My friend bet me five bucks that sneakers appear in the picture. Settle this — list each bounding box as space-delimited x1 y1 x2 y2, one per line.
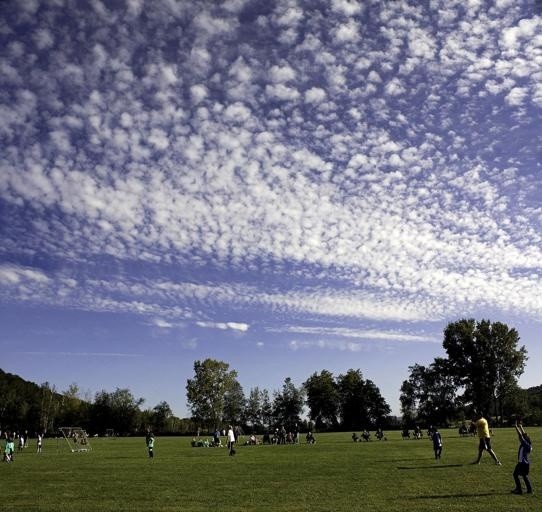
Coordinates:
511 488 523 496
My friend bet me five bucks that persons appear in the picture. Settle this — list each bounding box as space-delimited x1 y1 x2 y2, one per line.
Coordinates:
352 409 503 466
510 420 536 495
144 425 156 456
191 419 316 456
147 434 155 459
0 428 91 463
